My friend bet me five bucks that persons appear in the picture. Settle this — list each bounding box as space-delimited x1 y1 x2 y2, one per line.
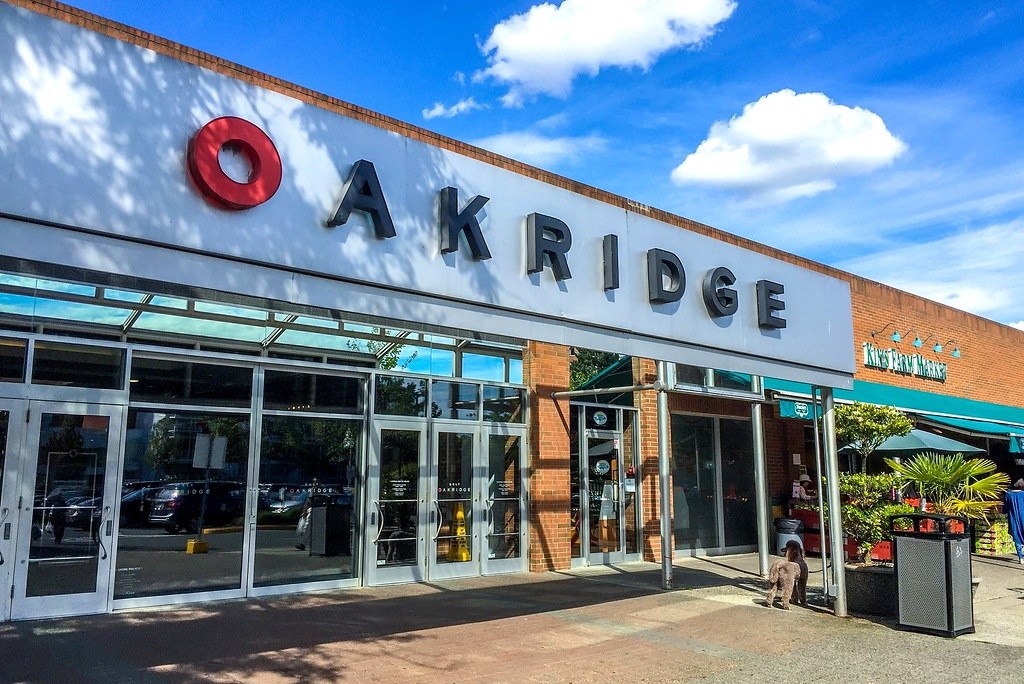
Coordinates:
798 474 818 500
1002 477 1024 564
43 488 67 545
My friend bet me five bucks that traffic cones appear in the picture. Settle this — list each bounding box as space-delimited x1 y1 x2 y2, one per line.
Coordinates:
445 501 471 562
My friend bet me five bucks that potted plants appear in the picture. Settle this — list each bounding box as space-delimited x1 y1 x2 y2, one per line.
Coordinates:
794 400 917 623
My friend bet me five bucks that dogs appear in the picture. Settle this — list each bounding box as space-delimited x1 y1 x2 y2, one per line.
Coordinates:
767 540 809 610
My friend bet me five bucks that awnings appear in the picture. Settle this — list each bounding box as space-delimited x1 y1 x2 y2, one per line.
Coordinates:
712 370 1024 454
573 355 638 448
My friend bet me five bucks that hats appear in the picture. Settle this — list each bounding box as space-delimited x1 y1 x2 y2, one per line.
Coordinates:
799 475 813 483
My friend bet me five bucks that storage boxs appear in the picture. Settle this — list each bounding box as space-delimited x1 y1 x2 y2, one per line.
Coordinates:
974 513 1024 557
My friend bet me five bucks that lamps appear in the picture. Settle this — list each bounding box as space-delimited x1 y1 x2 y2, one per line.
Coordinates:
941 340 961 358
872 323 901 343
922 334 942 353
894 329 923 349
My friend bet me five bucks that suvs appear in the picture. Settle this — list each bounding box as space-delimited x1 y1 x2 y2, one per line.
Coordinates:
147 479 270 534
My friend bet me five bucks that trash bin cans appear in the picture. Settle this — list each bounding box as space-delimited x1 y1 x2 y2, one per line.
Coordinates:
889 513 976 640
774 517 805 557
311 492 352 557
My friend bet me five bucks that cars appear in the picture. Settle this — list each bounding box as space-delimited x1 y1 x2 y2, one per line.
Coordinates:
260 482 355 527
31 479 172 529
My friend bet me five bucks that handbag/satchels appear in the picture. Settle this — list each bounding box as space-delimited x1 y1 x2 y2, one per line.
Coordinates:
297 516 306 536
45 521 53 533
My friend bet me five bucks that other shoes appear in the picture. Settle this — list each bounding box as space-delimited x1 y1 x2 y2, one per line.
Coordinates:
296 543 305 551
55 538 61 544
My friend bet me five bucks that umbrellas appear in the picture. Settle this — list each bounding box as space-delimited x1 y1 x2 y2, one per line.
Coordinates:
837 428 988 477
570 440 617 464
